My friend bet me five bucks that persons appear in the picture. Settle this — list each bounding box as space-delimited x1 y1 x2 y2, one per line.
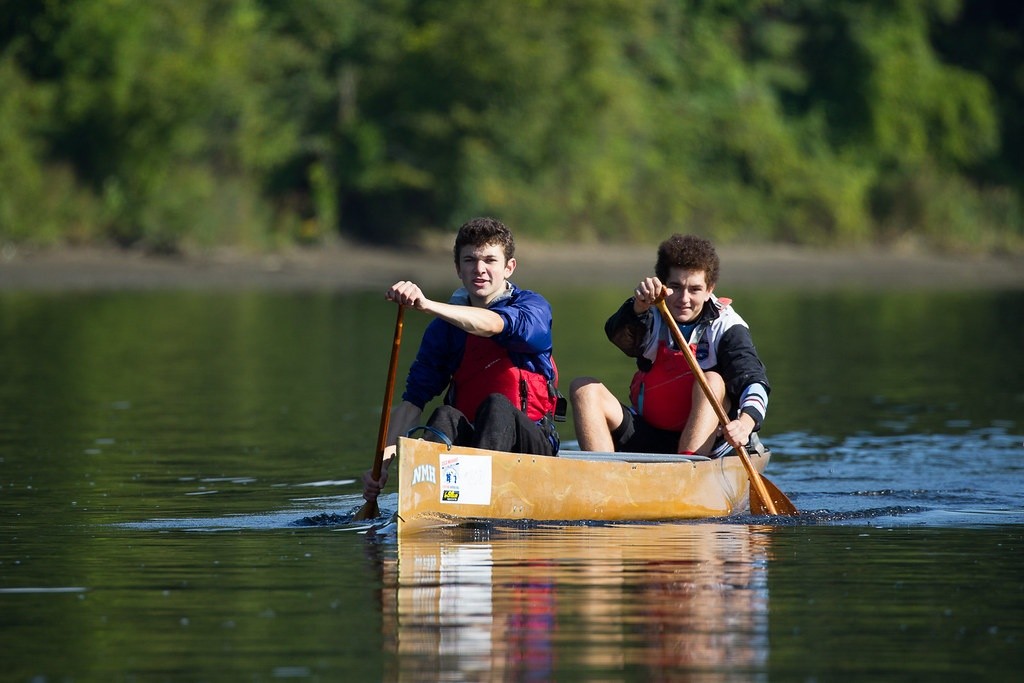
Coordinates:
361 218 561 503
568 233 771 462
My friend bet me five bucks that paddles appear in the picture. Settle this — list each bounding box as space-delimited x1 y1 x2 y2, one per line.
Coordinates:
647 285 798 514
354 298 404 518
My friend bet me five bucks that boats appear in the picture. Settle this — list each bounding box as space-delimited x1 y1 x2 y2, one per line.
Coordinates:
394 423 773 535
